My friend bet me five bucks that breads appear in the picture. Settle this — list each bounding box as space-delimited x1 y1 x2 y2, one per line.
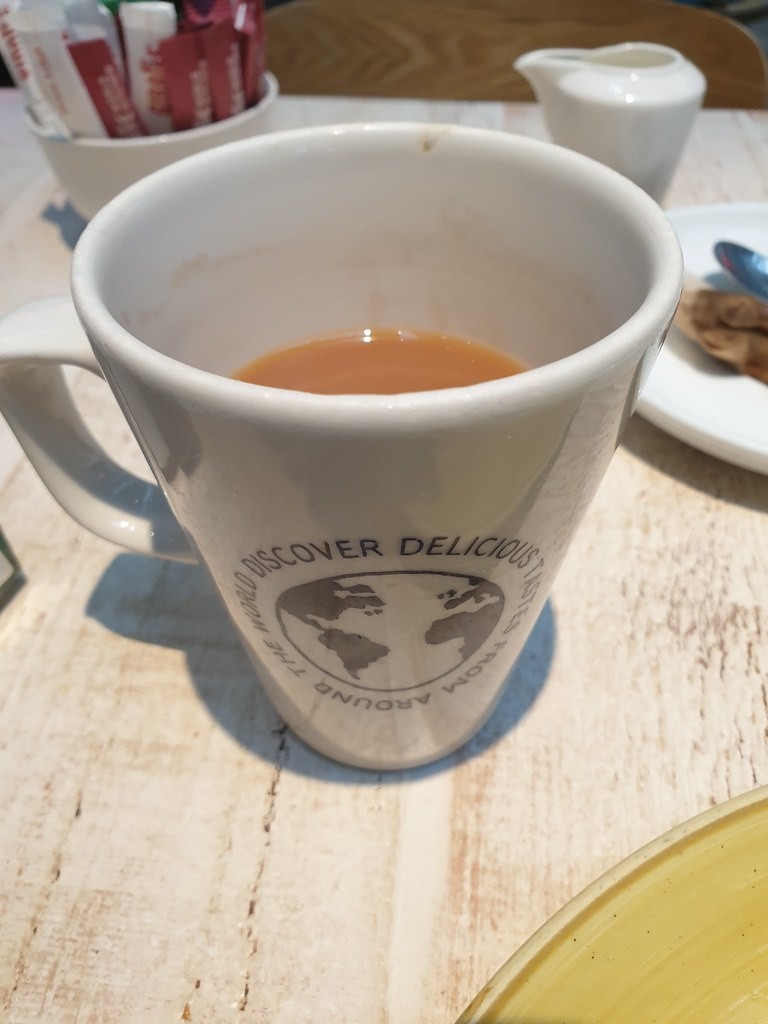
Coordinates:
672 276 768 382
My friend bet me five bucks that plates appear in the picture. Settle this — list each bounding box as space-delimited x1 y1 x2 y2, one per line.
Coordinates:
453 785 768 1024
635 200 768 476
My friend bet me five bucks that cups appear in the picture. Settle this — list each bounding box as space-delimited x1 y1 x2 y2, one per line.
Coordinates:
0 122 685 771
514 43 707 201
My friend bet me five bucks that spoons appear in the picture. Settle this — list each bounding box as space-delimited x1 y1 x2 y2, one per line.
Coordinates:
714 242 768 304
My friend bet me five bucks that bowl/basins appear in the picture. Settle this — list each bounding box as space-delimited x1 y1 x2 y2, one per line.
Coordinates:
22 71 279 221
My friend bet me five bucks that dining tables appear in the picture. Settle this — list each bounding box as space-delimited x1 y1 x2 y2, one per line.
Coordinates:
0 88 768 1024
